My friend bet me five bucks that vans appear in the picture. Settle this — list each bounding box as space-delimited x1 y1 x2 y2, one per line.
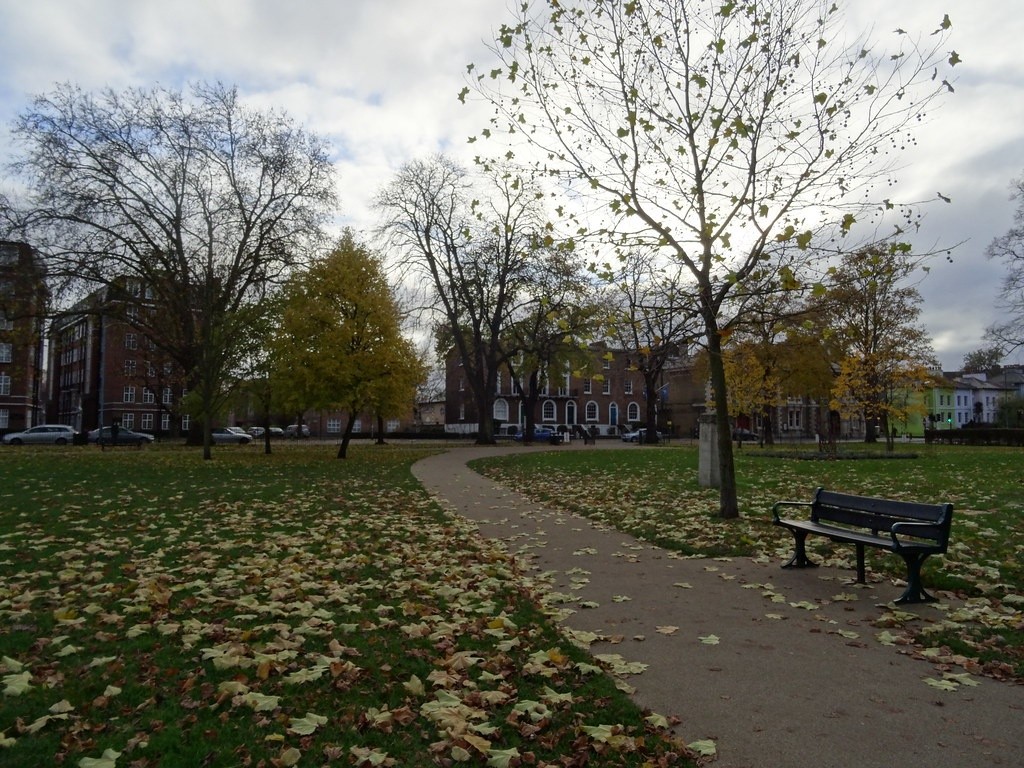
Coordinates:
284 424 311 438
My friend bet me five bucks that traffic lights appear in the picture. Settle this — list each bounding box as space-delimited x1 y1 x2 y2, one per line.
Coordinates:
947 412 952 423
929 412 933 421
937 413 941 421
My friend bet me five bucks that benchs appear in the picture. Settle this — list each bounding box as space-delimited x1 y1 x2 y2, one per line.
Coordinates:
775 487 956 605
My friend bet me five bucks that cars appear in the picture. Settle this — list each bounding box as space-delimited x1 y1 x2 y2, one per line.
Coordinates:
270 425 284 436
732 427 760 440
225 427 245 434
248 426 265 438
621 428 662 443
3 423 80 445
88 425 156 446
514 425 552 441
210 427 253 445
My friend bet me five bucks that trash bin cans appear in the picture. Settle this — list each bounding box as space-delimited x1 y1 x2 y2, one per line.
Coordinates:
639 429 646 444
